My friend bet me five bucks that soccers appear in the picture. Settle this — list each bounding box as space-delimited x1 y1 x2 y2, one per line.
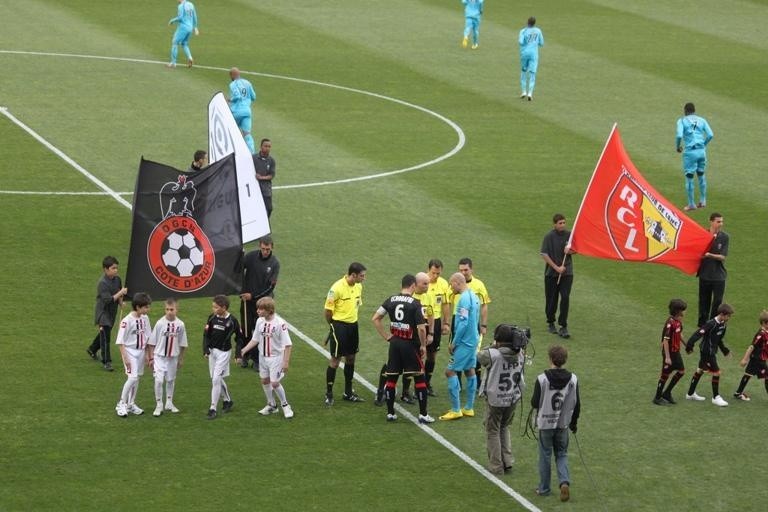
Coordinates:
161 229 205 278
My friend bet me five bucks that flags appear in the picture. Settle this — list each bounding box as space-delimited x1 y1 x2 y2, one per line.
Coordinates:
568 127 713 275
123 157 246 302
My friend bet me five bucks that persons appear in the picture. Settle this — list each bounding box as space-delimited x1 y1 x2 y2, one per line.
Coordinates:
477 323 526 473
539 214 577 338
696 213 730 328
461 0 484 50
164 0 200 68
324 262 368 406
88 235 294 419
518 17 544 100
652 300 768 407
531 345 581 502
227 68 256 154
252 139 275 218
185 150 208 228
674 103 712 211
371 258 492 424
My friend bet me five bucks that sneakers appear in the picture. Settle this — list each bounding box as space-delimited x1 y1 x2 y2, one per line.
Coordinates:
684 202 705 211
560 482 569 501
165 60 194 69
462 36 478 49
519 92 532 100
342 389 362 401
206 408 216 419
547 322 570 338
103 361 113 370
375 383 484 424
652 392 750 406
281 402 295 417
85 347 97 359
222 399 234 411
324 393 334 404
259 404 279 416
114 400 180 418
